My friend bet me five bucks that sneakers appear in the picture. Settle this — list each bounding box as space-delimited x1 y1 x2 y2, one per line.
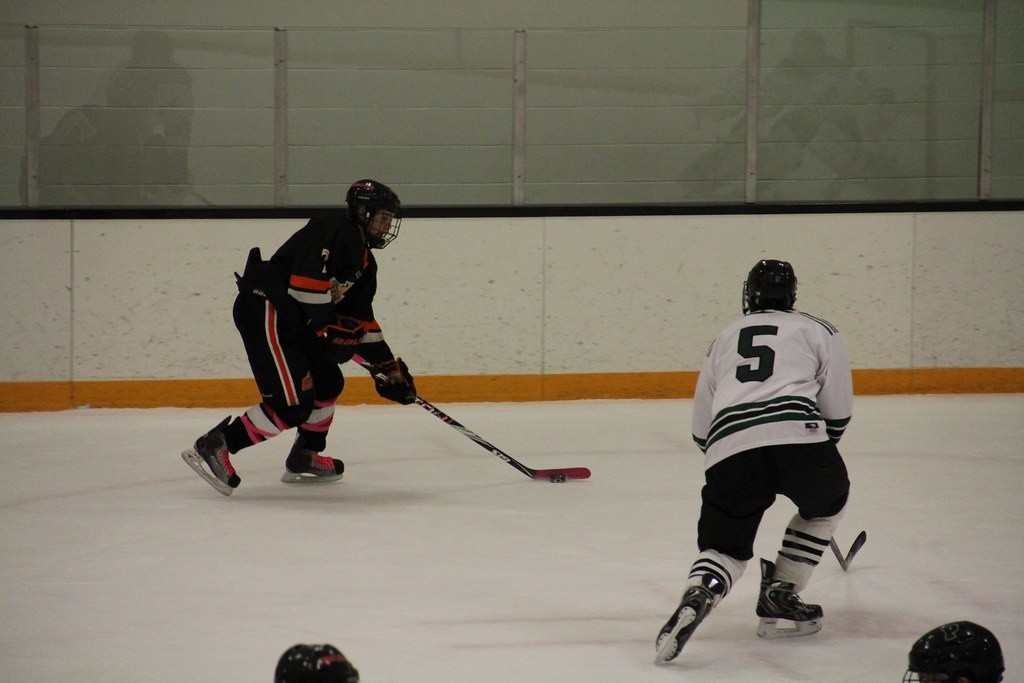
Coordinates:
756 557 824 640
653 572 728 665
281 448 345 483
181 415 241 496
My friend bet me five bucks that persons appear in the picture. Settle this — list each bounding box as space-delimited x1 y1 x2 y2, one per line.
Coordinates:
902 621 1006 683
652 259 853 658
180 178 417 493
272 643 360 683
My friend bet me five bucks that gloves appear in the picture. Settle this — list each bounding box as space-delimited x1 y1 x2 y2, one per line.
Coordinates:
316 318 367 363
375 357 416 405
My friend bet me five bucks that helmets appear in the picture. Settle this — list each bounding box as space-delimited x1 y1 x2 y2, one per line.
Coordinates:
345 179 403 249
742 259 798 315
902 621 1006 683
274 643 359 683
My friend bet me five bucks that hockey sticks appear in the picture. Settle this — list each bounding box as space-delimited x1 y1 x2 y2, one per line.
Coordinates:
829 530 867 572
351 353 592 480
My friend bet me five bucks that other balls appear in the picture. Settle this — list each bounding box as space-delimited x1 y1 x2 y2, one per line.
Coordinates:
551 473 565 484
